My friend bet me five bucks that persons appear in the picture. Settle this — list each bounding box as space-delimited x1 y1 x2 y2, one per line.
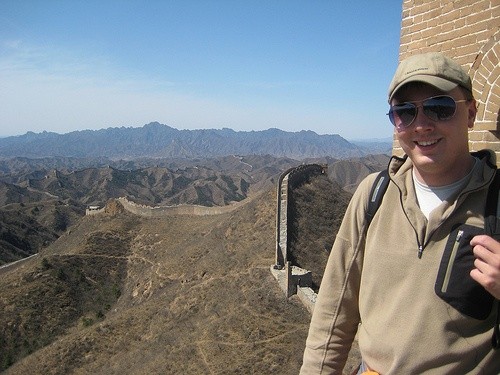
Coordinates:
298 52 500 375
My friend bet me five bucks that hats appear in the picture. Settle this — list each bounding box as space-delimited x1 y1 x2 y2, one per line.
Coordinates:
387 52 473 103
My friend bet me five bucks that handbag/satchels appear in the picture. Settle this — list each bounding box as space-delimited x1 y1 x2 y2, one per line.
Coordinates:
433 224 493 319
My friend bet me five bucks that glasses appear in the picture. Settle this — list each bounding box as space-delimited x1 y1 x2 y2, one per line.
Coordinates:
388 95 456 128
